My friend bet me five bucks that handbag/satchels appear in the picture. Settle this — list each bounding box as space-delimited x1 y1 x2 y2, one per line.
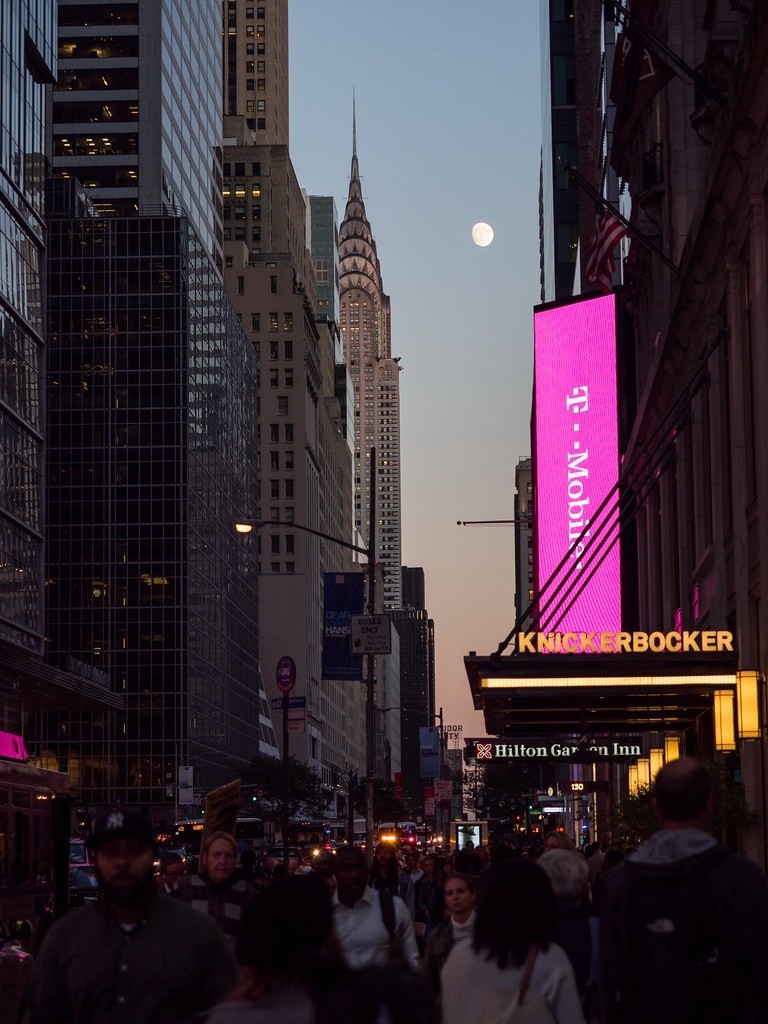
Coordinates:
483 943 555 1024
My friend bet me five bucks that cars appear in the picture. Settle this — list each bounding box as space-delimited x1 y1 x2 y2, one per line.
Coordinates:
0 798 453 1024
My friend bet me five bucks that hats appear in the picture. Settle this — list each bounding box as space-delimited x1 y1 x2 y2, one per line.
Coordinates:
86 808 155 848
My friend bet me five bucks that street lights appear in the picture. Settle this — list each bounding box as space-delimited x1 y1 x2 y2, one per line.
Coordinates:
229 446 379 868
376 707 444 832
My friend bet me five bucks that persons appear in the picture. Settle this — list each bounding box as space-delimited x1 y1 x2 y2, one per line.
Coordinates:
598 757 768 1024
27 808 237 1023
157 828 631 1024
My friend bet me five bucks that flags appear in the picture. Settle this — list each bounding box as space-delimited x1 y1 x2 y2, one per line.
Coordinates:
583 200 631 295
609 27 679 208
